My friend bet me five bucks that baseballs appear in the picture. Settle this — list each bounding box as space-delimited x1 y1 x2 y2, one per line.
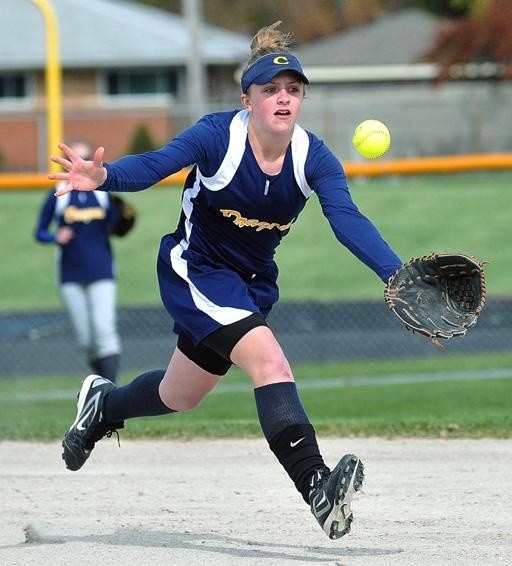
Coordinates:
353 119 391 158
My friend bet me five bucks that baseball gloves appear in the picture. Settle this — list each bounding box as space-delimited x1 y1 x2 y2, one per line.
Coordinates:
386 253 486 339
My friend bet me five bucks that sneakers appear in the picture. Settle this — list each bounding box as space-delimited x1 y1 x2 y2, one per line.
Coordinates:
61 373 125 472
307 454 366 540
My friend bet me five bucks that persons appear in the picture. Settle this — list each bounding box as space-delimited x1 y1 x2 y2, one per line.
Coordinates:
47 20 486 541
34 139 137 386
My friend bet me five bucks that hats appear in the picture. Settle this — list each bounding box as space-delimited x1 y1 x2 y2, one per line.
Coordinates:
239 51 309 95
